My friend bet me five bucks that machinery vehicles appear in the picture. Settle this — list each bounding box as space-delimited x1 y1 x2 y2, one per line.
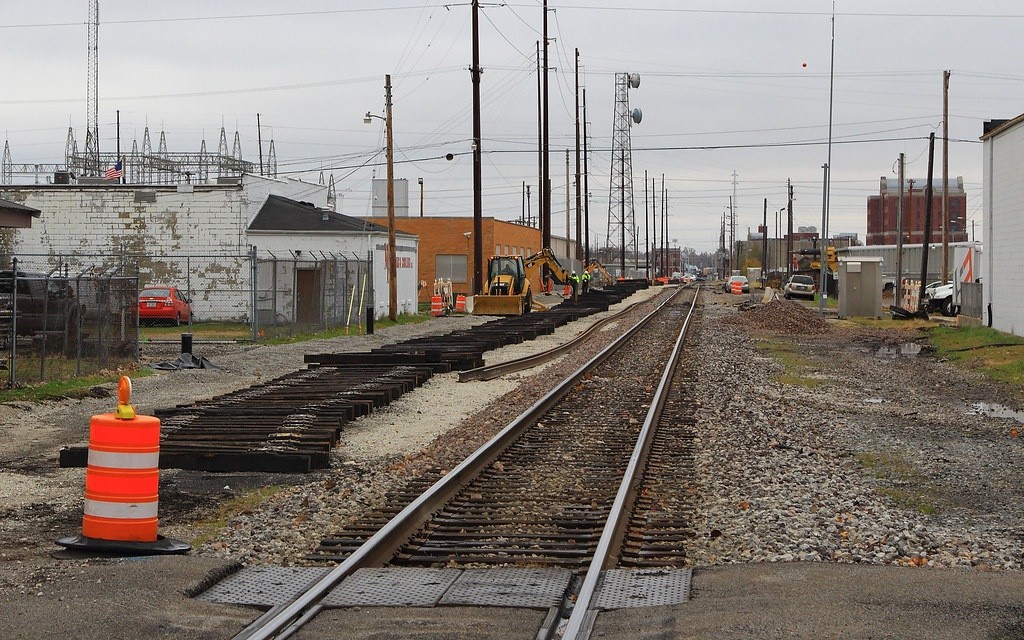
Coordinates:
470 247 570 316
584 258 614 284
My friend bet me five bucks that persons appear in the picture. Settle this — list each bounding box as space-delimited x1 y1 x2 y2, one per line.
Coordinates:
582 271 591 292
793 258 798 271
570 271 580 283
503 264 514 274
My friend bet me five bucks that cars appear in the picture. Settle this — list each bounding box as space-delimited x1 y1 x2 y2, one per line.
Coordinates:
725 276 750 293
924 280 961 317
783 275 816 301
130 284 194 325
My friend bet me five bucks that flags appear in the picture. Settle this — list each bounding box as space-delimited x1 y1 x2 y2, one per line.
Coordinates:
104 160 122 180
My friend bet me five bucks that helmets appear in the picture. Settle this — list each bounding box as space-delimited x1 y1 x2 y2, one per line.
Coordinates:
584 271 588 274
572 271 575 274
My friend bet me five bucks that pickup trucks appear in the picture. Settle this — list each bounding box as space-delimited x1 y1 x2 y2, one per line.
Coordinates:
0 270 86 350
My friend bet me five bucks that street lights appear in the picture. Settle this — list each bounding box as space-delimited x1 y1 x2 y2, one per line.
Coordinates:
566 191 593 281
774 208 786 278
363 74 398 323
